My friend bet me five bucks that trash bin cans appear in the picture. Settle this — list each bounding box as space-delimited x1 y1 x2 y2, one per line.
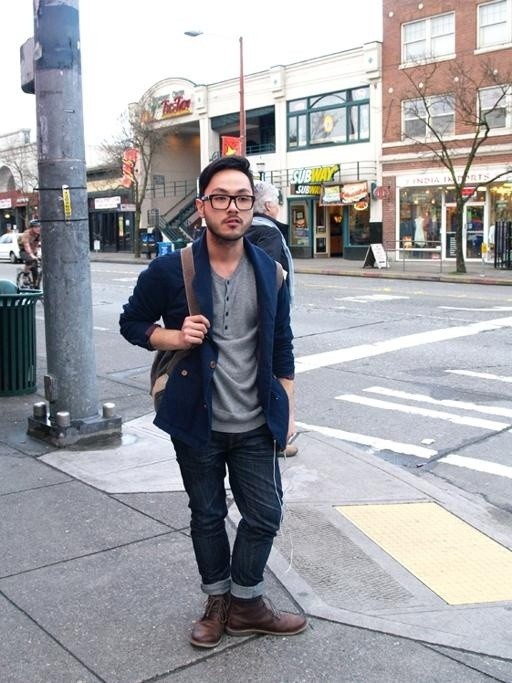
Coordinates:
0 279 45 397
157 241 187 257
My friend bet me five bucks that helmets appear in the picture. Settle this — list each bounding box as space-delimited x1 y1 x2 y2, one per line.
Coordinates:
29 218 40 227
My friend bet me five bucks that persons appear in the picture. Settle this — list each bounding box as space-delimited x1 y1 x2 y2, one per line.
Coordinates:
16 217 41 287
112 154 311 648
243 178 294 300
411 215 427 247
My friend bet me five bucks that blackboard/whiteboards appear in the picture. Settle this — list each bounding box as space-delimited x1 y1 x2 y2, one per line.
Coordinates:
371 244 389 267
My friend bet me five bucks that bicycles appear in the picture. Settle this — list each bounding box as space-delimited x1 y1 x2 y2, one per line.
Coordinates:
16 257 44 304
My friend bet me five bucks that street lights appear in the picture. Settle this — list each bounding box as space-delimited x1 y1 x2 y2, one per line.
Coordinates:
183 29 246 159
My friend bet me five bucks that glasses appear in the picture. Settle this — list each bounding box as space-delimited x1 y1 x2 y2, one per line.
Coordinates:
201 193 255 211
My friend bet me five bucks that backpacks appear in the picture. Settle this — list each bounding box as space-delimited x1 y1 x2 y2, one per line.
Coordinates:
150 245 284 411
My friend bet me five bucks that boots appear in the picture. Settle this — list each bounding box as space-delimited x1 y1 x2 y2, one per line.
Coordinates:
225 594 309 636
190 590 230 648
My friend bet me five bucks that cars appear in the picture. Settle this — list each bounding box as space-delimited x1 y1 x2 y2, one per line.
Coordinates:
0 232 42 264
